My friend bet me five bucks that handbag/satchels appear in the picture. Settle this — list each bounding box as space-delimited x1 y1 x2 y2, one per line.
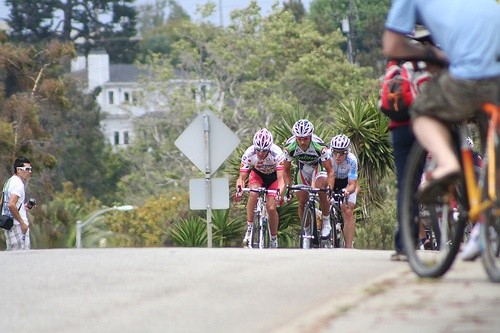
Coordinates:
0 215 13 230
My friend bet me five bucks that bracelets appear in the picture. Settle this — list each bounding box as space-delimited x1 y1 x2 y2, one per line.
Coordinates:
424 41 436 62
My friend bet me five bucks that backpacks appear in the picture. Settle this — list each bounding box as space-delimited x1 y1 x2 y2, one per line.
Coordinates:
378 59 433 121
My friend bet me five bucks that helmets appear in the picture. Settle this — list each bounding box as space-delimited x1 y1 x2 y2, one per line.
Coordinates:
291 119 314 137
252 128 273 150
331 133 350 151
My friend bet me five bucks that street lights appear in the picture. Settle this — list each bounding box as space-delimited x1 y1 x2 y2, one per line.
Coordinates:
75 205 133 249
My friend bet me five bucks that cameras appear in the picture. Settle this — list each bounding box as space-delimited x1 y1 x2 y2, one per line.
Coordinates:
27 199 36 209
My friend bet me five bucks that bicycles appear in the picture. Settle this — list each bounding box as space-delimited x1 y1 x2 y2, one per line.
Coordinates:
236 186 278 249
397 53 500 282
286 184 349 250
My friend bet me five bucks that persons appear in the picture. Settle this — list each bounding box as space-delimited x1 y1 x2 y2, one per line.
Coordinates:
282 119 334 249
377 0 500 261
327 133 360 249
0 156 37 251
235 127 286 249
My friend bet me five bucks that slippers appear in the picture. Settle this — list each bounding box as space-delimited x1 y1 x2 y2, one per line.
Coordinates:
415 169 461 203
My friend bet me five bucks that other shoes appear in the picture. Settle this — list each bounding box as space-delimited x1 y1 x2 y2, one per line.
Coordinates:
389 251 407 261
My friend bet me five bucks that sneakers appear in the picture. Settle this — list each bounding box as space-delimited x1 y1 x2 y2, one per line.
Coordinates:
271 235 279 248
459 227 497 262
243 227 252 244
321 224 331 237
302 237 310 248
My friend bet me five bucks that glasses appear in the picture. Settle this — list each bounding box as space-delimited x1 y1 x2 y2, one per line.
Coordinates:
332 150 348 155
256 149 268 153
296 138 310 141
17 167 32 171
